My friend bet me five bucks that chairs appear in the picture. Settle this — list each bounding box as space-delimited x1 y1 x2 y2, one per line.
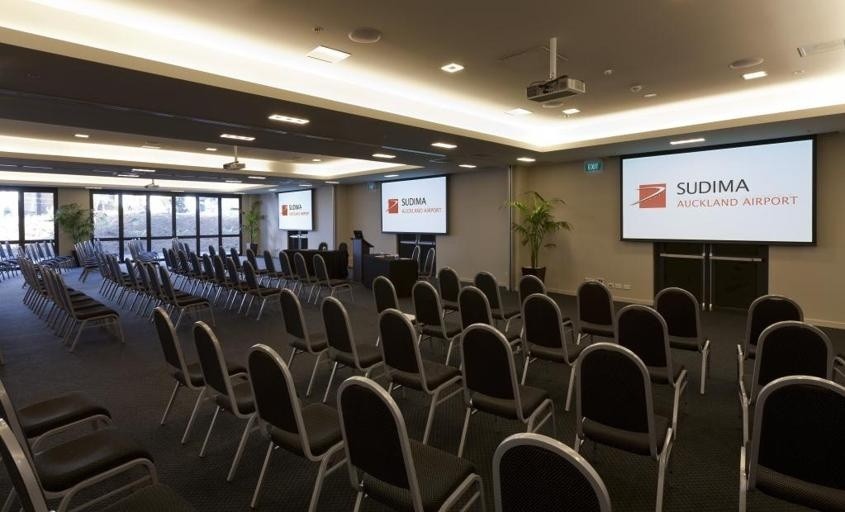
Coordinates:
189 317 279 483
333 371 489 509
566 341 679 509
278 263 844 469
2 233 358 353
736 373 843 512
490 429 614 507
0 370 170 512
150 303 249 446
242 341 347 509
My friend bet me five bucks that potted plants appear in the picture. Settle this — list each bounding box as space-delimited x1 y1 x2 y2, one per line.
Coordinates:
231 200 269 257
49 201 99 267
501 190 574 284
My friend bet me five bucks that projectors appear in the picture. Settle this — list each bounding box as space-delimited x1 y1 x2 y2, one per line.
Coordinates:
526 78 585 103
145 185 159 190
223 162 246 171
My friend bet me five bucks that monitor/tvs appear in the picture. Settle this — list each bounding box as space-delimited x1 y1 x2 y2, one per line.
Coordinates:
354 230 363 239
